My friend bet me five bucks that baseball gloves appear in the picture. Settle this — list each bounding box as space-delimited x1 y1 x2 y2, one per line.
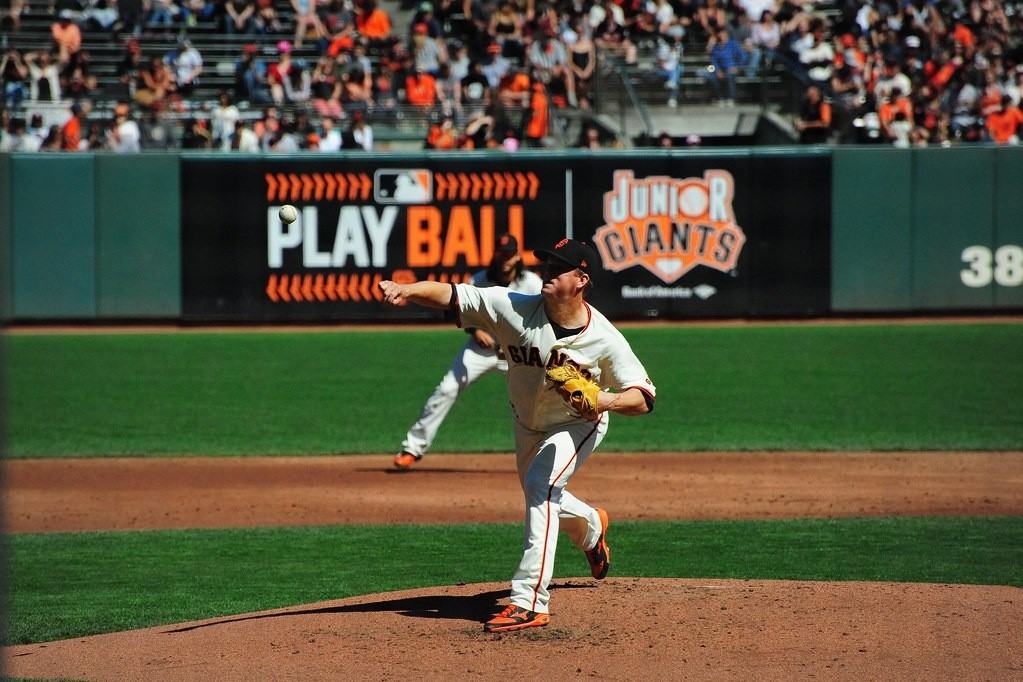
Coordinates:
546 363 602 423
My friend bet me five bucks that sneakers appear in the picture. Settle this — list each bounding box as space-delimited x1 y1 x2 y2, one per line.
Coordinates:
484 604 550 633
394 450 415 469
584 508 610 580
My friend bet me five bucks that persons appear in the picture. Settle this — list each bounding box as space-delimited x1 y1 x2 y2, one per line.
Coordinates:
378 239 658 632
0 0 1023 153
393 234 545 468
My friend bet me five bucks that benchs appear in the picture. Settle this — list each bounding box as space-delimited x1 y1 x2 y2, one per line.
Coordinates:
0 0 801 140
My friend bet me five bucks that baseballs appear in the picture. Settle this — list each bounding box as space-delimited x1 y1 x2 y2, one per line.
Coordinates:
279 205 298 224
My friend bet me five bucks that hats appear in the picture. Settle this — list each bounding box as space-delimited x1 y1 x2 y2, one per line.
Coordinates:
534 239 597 282
495 234 518 252
